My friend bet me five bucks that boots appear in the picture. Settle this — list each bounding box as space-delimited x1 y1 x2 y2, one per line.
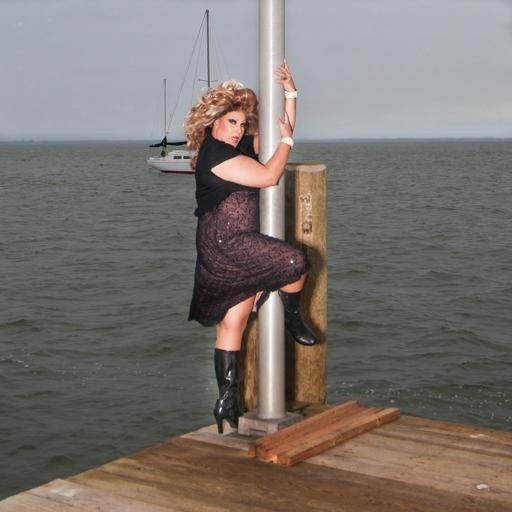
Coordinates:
280 288 318 346
212 347 246 435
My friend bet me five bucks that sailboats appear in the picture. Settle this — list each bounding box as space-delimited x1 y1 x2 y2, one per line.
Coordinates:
147 9 218 176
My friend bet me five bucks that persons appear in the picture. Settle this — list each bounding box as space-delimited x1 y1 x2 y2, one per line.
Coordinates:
183 60 318 435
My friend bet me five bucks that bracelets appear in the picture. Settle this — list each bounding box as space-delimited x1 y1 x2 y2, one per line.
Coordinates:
284 90 298 100
281 137 295 146
